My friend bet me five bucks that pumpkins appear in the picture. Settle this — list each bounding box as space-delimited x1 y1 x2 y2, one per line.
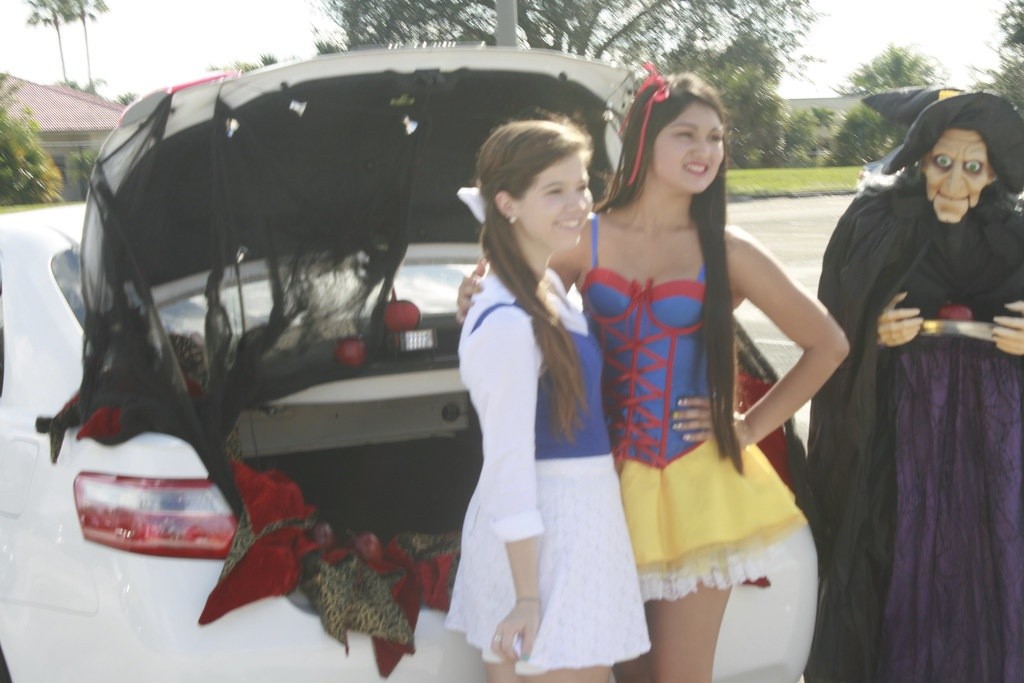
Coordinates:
385 289 420 332
336 335 369 368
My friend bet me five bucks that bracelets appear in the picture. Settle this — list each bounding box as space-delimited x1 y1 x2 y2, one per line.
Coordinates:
516 597 541 604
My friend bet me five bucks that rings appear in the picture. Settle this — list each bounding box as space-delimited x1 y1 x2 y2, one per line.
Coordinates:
493 635 502 642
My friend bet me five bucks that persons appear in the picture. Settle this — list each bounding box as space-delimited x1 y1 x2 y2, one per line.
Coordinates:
455 71 849 683
440 120 652 683
801 85 1024 683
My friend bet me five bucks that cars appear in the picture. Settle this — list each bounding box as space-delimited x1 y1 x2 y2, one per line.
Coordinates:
858 141 907 194
0 47 812 682
